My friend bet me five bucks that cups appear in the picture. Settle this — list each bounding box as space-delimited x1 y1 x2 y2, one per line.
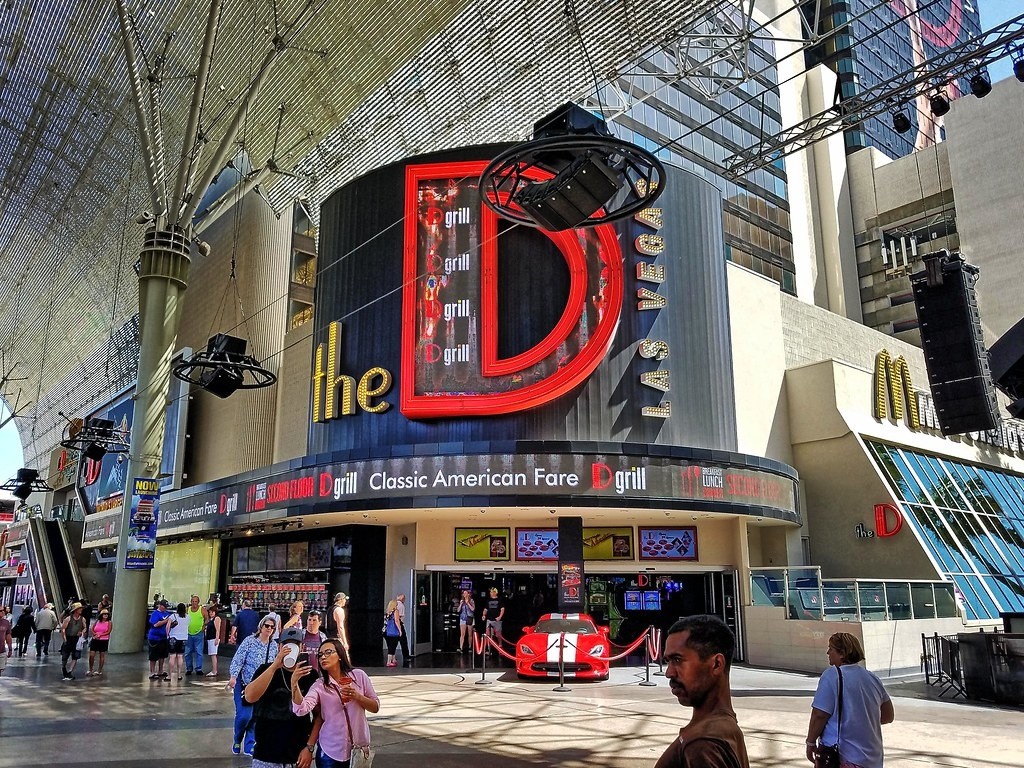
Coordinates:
282 643 299 668
339 677 352 703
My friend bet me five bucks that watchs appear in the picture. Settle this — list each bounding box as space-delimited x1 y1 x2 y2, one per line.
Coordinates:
306 743 314 753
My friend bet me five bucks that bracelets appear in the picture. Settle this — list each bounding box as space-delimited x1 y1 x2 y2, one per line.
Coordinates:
9 649 12 651
806 739 816 747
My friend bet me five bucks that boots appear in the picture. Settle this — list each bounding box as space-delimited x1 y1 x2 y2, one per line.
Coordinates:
386 654 396 666
391 655 398 664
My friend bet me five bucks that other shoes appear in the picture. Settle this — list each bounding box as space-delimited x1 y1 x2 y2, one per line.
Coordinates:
62 674 76 681
36 651 48 657
85 670 103 675
485 649 492 655
499 647 505 655
156 672 168 677
206 671 217 677
185 671 204 676
149 673 159 679
457 648 463 653
402 655 415 660
469 648 472 652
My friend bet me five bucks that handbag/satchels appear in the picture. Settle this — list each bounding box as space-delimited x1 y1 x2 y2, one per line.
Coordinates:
814 744 841 768
170 613 178 628
76 632 85 650
466 616 474 626
387 620 400 637
349 744 375 768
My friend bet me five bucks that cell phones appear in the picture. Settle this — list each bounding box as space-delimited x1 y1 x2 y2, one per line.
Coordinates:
299 652 310 667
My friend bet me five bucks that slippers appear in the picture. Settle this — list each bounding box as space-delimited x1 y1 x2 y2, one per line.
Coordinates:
178 676 182 680
163 676 171 681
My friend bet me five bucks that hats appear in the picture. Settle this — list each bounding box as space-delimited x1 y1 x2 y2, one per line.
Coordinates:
100 609 110 615
160 599 171 607
279 628 302 641
102 594 109 598
334 592 350 602
70 602 84 613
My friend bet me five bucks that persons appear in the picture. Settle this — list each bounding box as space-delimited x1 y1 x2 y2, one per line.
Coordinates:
257 602 281 653
14 605 39 658
282 601 304 630
506 586 518 610
532 588 544 608
147 593 222 680
33 603 62 656
654 615 750 768
448 586 475 652
0 604 14 677
482 586 505 655
245 628 325 768
290 638 381 768
384 593 414 667
302 610 329 676
60 594 113 678
325 592 351 666
807 632 894 768
227 616 279 756
230 600 259 650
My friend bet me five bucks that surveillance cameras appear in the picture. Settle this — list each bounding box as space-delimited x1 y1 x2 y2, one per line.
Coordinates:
118 456 124 464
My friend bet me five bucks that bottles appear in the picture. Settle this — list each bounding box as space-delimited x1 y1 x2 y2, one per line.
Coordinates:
422 595 425 604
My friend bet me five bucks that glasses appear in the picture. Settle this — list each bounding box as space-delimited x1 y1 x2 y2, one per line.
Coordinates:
0 609 5 612
264 623 275 629
318 649 338 658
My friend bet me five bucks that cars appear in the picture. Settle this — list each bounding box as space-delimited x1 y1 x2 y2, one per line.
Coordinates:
515 613 610 680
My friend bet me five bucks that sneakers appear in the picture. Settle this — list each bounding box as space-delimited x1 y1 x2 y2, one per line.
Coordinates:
231 741 254 757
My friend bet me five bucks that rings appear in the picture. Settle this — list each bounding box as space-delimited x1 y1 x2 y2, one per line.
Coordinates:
349 692 352 696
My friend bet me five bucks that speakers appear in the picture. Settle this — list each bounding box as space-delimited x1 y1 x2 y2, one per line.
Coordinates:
13 469 38 501
200 334 246 400
512 102 625 232
82 419 116 462
910 263 1003 437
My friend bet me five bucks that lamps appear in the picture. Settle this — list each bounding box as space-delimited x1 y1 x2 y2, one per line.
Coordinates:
890 240 898 271
103 548 107 555
260 523 266 533
477 0 668 235
177 538 183 543
170 251 276 400
900 236 909 267
200 532 206 541
156 540 163 547
882 247 889 265
929 86 951 118
297 518 304 529
970 66 993 99
911 236 918 257
0 427 55 500
90 548 95 555
892 105 912 134
228 528 234 537
168 539 171 544
190 534 194 542
113 545 117 552
57 360 131 462
1013 48 1024 83
281 520 288 530
985 316 1024 421
215 530 221 539
246 525 253 535
